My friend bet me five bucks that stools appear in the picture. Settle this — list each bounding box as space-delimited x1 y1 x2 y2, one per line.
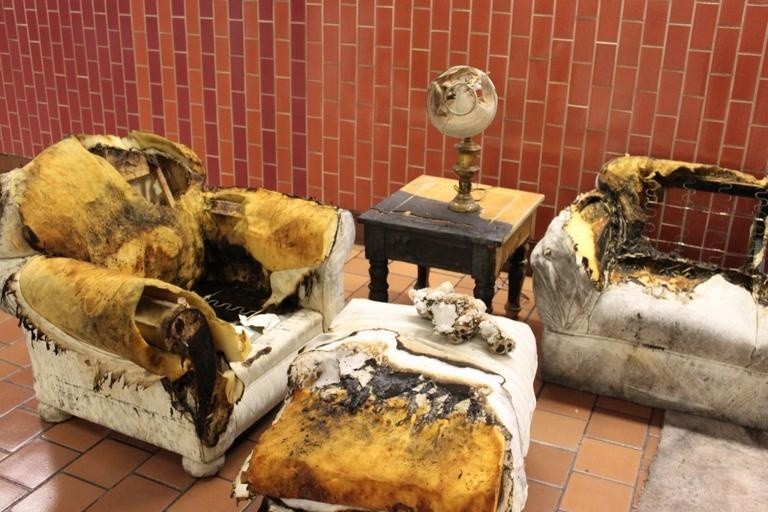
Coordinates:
234 298 540 510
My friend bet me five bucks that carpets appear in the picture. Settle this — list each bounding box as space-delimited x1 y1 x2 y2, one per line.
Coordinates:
629 410 767 510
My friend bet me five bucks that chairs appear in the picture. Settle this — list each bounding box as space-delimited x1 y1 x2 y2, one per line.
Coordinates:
0 131 357 477
529 154 767 437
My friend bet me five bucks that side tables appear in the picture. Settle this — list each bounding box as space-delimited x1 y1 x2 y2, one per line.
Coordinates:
357 171 546 316
